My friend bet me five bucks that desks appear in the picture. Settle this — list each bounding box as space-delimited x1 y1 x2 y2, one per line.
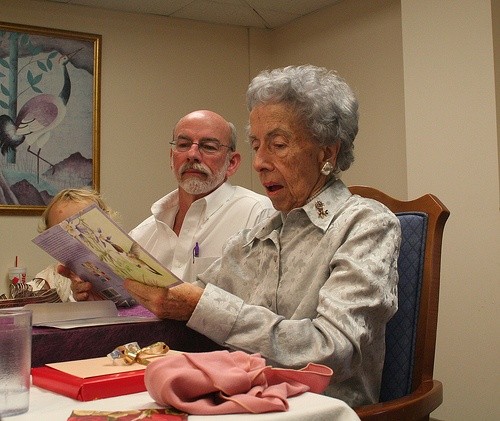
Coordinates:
0 349 361 421
0 317 221 375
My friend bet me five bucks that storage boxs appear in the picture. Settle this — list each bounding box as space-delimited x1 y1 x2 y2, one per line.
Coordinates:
30 351 187 402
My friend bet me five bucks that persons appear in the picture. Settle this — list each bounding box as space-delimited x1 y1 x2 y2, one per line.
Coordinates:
57 63 402 409
25 187 123 303
128 110 278 283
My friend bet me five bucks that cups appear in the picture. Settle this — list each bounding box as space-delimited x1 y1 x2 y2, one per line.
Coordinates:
8 266 27 284
0 308 33 417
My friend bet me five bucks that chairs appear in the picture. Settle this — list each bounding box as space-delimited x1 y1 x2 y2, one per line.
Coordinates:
346 186 450 421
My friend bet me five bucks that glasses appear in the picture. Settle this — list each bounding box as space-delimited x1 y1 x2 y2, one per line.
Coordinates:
168 137 233 153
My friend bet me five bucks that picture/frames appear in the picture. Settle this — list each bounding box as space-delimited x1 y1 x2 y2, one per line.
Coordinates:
0 21 102 209
